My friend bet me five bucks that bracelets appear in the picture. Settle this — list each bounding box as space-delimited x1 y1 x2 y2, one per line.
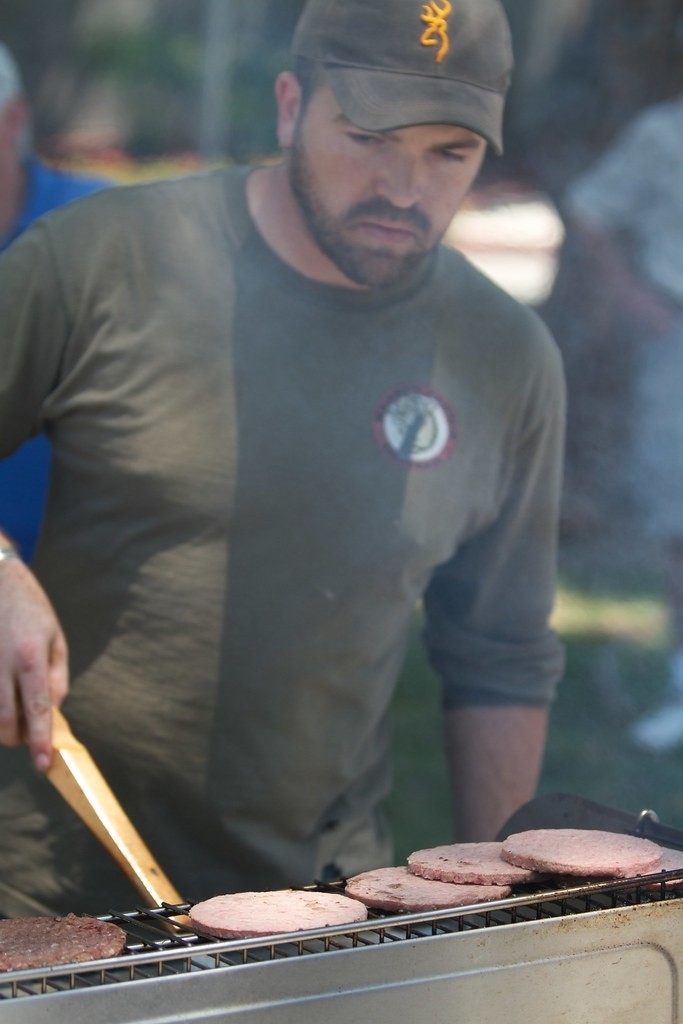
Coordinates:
0 549 20 562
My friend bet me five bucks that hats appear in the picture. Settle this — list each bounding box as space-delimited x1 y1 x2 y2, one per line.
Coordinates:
291 0 514 156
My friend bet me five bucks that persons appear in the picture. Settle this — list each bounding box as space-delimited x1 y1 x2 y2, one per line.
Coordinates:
570 0 683 747
0 0 570 925
